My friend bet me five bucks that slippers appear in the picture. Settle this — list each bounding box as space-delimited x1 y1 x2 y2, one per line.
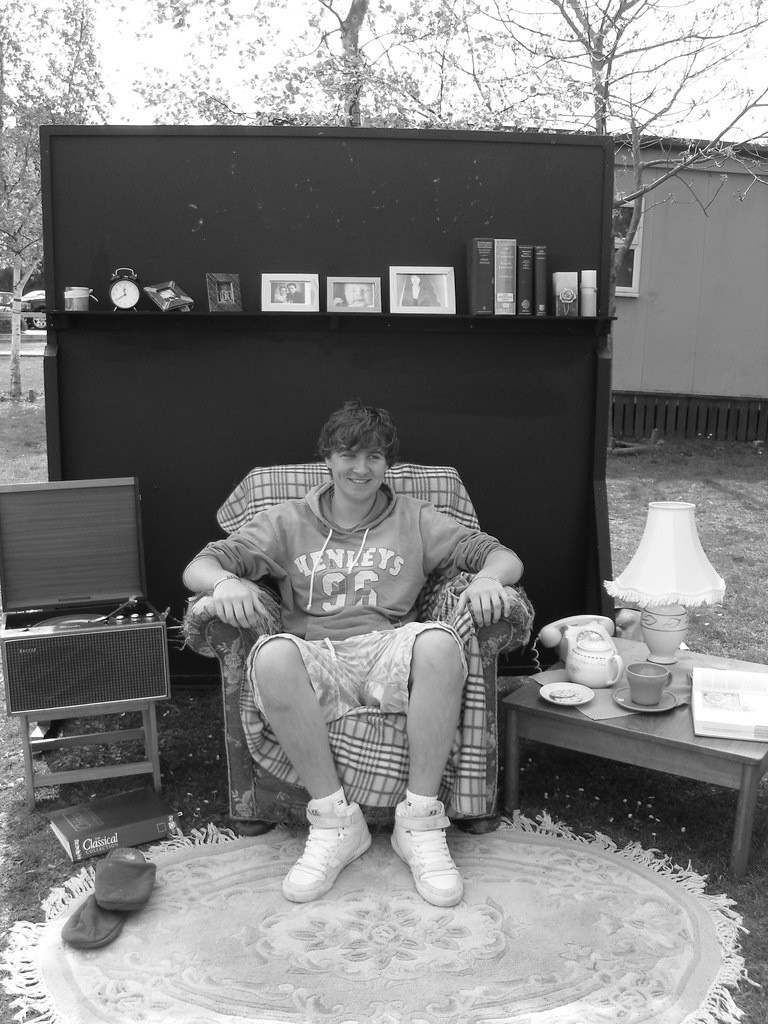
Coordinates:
62 890 127 948
94 847 157 911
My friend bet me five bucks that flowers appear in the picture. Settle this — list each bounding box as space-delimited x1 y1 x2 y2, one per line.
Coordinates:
560 289 576 303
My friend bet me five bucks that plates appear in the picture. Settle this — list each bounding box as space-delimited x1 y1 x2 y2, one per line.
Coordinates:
539 682 594 706
613 686 678 712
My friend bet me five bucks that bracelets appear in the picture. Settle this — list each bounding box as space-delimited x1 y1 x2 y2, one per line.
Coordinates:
213 575 241 588
472 575 500 583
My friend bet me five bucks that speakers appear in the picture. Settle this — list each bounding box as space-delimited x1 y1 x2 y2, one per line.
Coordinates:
0 476 173 716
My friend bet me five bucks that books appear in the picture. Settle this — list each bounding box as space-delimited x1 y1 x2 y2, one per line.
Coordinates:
690 667 768 743
466 237 547 316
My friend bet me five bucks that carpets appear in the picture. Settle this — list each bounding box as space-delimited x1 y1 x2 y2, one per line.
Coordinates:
1 809 765 1024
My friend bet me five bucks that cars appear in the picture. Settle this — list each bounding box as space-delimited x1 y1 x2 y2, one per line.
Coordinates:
0 290 46 334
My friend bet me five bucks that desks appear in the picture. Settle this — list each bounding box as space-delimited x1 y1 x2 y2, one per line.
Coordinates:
20 701 163 811
502 636 768 877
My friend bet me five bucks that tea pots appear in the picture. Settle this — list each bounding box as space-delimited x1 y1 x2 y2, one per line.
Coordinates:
565 636 624 688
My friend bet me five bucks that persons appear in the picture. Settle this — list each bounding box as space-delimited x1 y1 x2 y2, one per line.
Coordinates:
183 399 524 907
274 283 302 303
333 283 373 307
403 275 429 306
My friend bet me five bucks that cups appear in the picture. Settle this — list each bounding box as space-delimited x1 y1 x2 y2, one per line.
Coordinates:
64 287 98 311
626 663 673 705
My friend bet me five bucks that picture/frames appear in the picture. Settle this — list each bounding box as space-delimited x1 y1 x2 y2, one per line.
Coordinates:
206 273 243 313
142 280 194 312
327 277 382 313
388 266 456 314
261 273 320 312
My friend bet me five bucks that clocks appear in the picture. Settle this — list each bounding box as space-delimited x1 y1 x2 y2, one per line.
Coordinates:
108 268 141 311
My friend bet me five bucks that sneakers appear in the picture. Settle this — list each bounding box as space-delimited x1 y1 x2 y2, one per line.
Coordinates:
283 799 373 901
390 800 462 906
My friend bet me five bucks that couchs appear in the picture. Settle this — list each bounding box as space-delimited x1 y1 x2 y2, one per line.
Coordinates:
184 462 536 835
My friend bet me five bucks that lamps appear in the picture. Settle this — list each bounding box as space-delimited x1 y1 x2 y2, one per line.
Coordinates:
602 502 727 665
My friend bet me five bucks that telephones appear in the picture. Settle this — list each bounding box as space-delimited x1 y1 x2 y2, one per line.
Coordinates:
539 613 616 661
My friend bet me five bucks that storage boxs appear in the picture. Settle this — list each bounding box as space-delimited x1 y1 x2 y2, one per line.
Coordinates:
0 477 171 718
